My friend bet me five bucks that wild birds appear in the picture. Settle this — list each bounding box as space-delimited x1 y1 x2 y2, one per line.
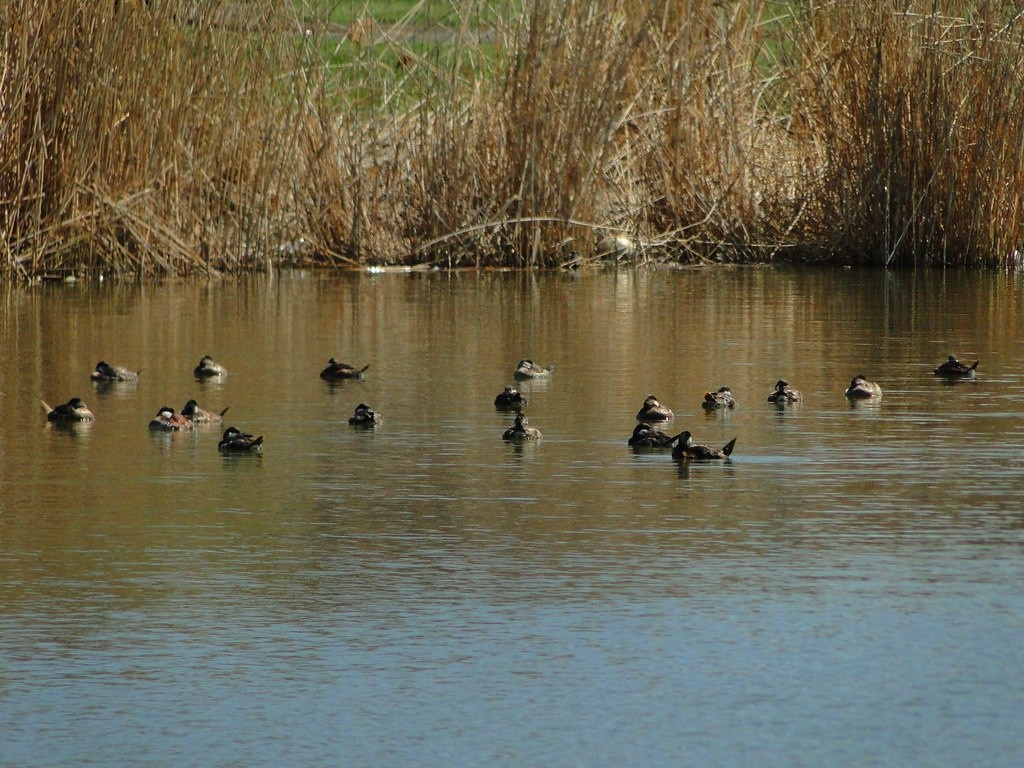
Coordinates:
493 358 555 444
347 402 385 432
626 384 739 467
147 399 265 459
843 373 883 403
933 352 980 382
88 359 146 386
38 396 94 435
766 378 803 407
319 356 373 393
191 353 229 384
